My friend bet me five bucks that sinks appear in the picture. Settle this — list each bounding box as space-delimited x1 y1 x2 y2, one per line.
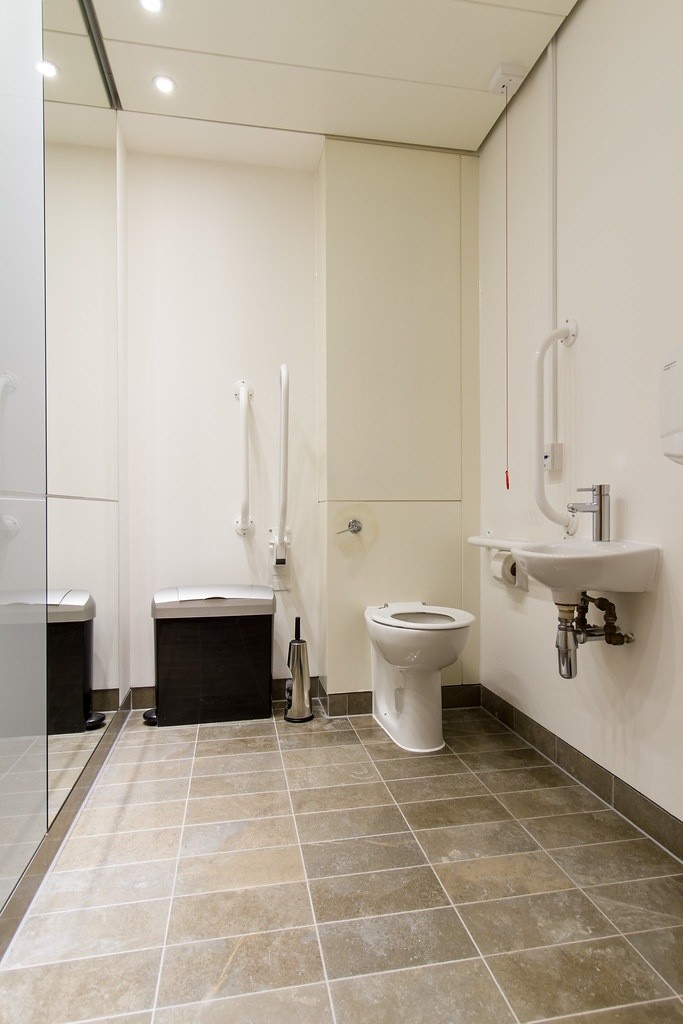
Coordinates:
467 535 662 606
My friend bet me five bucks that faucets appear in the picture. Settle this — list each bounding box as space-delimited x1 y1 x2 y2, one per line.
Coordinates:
566 483 611 543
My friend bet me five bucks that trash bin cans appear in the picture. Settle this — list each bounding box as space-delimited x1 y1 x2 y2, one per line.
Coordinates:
1 587 106 736
143 585 277 726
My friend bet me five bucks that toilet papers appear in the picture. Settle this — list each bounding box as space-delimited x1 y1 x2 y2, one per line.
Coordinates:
489 550 529 593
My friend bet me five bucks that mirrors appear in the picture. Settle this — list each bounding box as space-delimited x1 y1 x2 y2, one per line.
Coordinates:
42 0 120 832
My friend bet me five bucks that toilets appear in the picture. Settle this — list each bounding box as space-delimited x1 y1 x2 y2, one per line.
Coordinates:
364 601 477 753
